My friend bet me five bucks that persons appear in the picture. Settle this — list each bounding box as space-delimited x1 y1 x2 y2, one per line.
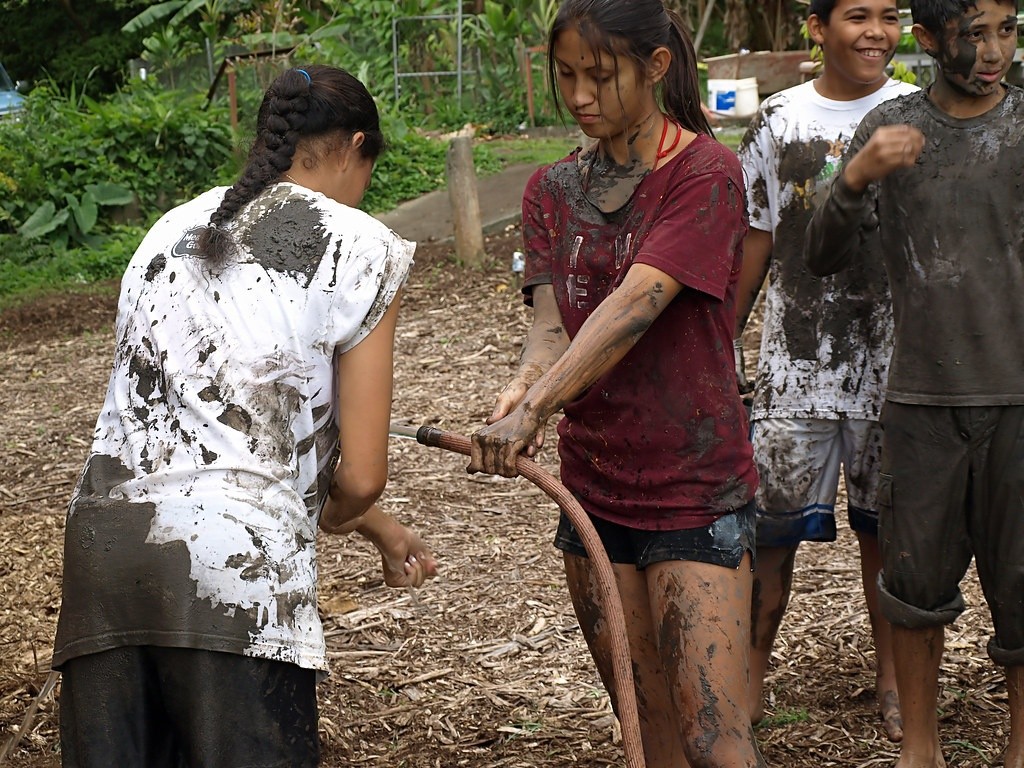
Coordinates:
55 66 439 768
733 1 1023 768
465 1 768 768
